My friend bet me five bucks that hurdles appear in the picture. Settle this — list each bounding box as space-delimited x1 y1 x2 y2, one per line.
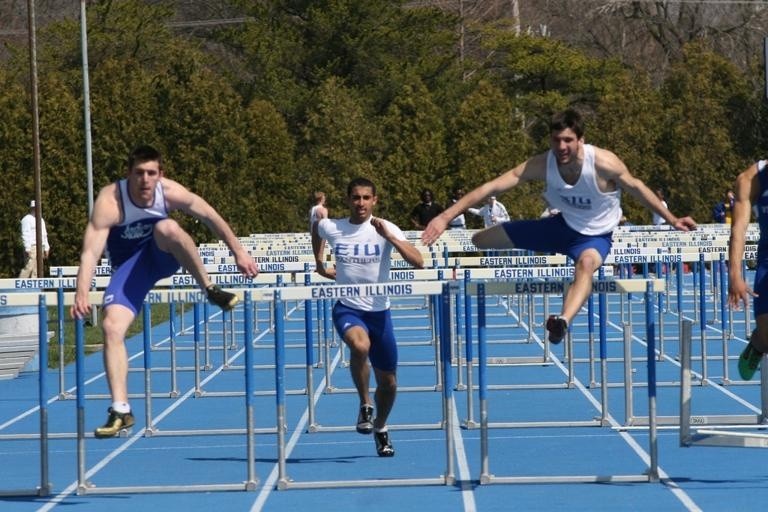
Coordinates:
0 223 764 499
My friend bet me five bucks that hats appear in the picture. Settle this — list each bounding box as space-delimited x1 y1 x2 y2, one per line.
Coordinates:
30 200 36 207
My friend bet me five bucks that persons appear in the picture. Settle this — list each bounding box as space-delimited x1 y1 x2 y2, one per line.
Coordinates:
308 191 328 234
726 157 768 384
409 189 444 230
466 196 509 227
651 189 667 225
18 200 51 278
619 215 637 232
312 177 422 459
712 189 734 222
424 108 700 344
445 188 466 230
71 145 259 438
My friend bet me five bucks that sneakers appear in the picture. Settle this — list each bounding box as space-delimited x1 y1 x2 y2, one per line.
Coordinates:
357 405 374 433
547 315 567 343
373 419 394 456
206 286 238 311
96 407 134 437
738 343 762 380
